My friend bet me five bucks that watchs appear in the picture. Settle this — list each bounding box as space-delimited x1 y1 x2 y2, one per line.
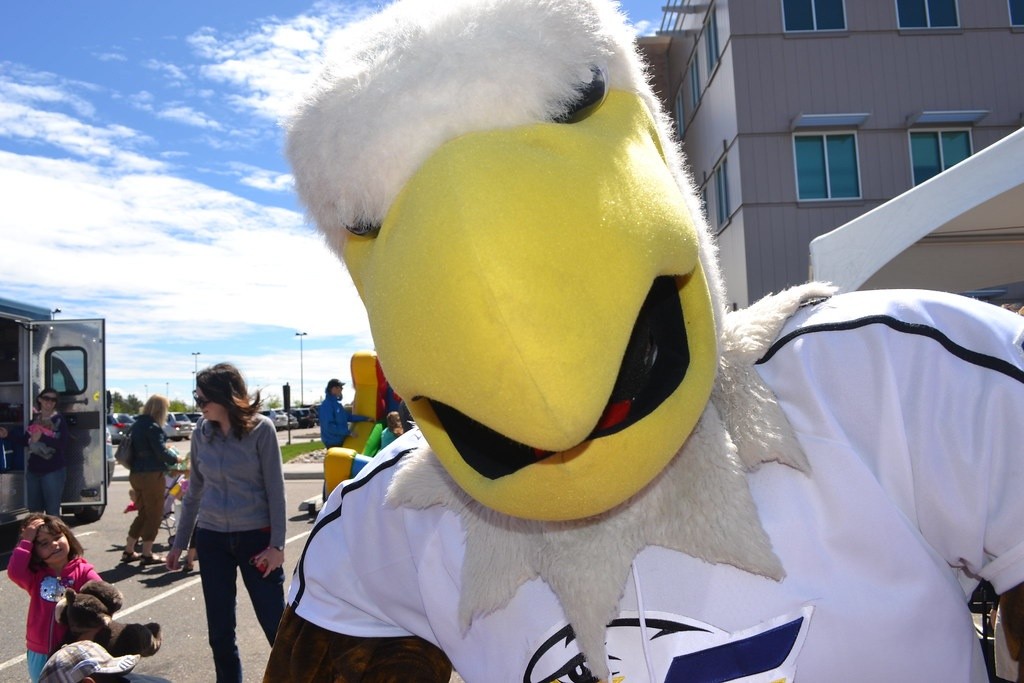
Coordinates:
274 545 284 551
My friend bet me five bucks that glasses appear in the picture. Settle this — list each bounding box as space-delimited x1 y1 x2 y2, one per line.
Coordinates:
40 395 58 403
192 390 213 410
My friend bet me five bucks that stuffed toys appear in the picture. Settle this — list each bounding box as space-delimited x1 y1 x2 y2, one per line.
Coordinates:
262 0 1024 683
55 580 161 658
28 418 57 459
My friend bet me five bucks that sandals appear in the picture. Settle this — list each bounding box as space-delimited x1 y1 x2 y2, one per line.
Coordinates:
140 554 162 565
120 551 142 562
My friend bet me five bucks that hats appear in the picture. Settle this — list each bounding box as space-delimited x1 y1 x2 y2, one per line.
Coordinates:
328 379 345 386
38 640 141 683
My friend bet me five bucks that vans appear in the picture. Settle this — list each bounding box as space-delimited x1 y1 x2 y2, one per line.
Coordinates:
0 296 114 523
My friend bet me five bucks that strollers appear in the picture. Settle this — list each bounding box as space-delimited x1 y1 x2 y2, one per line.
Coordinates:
127 451 193 547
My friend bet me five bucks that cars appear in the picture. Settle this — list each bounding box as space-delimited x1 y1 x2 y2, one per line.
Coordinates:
284 407 319 429
161 412 193 441
106 413 137 439
183 411 204 439
259 409 288 432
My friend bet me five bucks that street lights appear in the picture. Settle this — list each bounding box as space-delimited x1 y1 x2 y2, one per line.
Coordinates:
296 332 307 406
192 352 200 406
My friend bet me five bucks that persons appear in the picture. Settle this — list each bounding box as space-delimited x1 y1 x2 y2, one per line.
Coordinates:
22 388 66 516
7 514 103 683
165 362 287 683
318 379 404 504
122 395 198 571
